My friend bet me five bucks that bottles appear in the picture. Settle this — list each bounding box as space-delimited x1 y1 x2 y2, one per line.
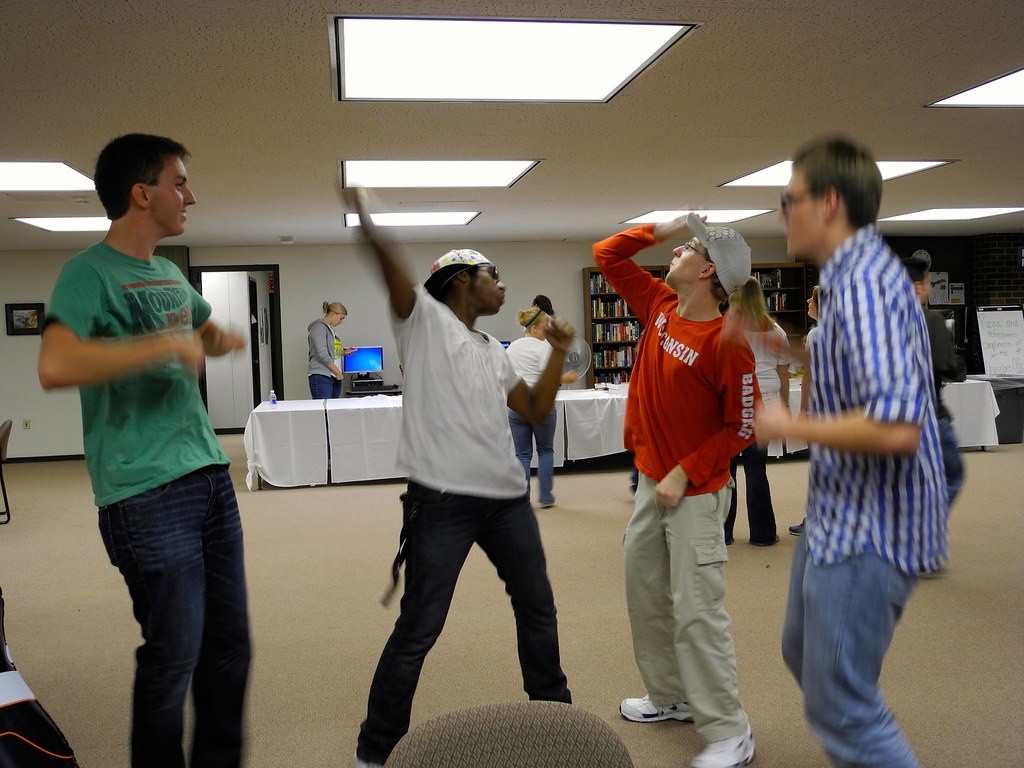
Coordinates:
269 390 277 408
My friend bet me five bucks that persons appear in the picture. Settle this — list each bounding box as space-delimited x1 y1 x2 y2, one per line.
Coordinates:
307 301 355 400
592 212 765 768
532 295 554 315
37 134 252 768
754 135 952 768
506 306 579 507
788 286 818 536
339 183 576 768
724 277 791 545
903 258 967 579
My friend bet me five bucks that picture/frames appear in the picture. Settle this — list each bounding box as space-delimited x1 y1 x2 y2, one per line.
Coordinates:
5 303 45 335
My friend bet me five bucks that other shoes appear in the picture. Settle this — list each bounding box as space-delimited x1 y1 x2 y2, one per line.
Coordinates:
788 516 808 536
922 565 951 579
539 498 556 507
775 534 780 541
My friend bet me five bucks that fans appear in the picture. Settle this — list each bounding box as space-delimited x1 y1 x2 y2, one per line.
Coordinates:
560 334 593 391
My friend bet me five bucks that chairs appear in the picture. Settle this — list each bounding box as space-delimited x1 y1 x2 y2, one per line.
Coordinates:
0 419 13 524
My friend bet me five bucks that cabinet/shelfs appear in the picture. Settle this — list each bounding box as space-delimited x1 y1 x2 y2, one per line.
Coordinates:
581 262 808 390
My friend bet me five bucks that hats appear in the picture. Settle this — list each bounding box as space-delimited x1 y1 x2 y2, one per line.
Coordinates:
686 212 751 296
422 248 491 287
533 295 554 315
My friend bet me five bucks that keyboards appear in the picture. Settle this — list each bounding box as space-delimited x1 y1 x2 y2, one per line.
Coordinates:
352 382 383 391
354 385 393 391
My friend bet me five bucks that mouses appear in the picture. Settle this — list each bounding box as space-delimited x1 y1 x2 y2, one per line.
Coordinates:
393 384 398 388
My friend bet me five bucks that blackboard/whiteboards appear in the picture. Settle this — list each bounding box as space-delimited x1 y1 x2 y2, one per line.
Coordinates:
977 306 1024 375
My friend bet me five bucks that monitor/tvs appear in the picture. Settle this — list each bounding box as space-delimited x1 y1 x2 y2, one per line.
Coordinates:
342 346 383 380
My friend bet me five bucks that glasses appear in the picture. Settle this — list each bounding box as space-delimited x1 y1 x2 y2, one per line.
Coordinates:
473 264 500 284
779 191 828 216
683 242 712 262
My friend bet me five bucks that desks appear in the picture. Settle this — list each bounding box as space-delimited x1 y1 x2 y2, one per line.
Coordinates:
245 379 1001 490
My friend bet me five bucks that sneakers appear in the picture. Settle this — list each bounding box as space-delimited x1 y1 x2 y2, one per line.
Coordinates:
619 692 695 724
689 721 755 768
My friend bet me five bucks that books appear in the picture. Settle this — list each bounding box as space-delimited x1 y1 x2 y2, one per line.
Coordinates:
753 269 784 311
590 274 639 385
